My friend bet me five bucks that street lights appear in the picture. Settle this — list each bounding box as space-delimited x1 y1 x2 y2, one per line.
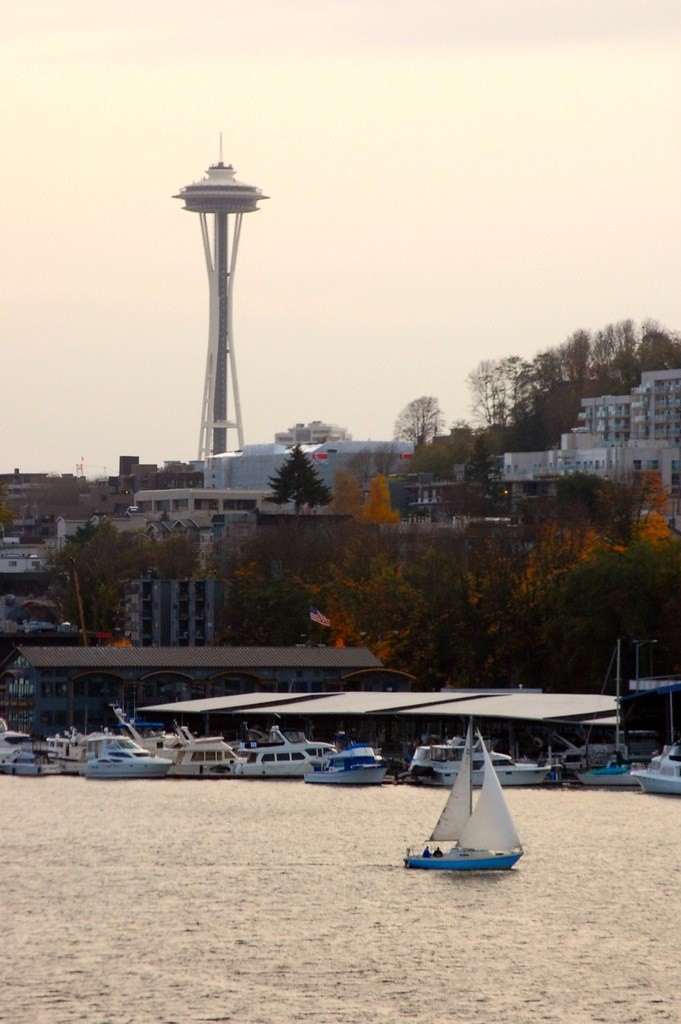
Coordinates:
635 640 657 693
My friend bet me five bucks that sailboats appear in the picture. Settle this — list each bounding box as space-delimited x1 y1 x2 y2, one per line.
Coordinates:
402 716 524 871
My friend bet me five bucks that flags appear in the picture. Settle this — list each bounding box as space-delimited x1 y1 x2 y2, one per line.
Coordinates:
309 605 331 627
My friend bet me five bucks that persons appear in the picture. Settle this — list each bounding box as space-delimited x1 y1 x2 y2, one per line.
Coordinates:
423 847 430 858
433 847 443 858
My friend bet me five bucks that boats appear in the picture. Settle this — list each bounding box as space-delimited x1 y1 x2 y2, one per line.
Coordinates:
576 762 647 787
1 639 659 793
630 739 681 794
